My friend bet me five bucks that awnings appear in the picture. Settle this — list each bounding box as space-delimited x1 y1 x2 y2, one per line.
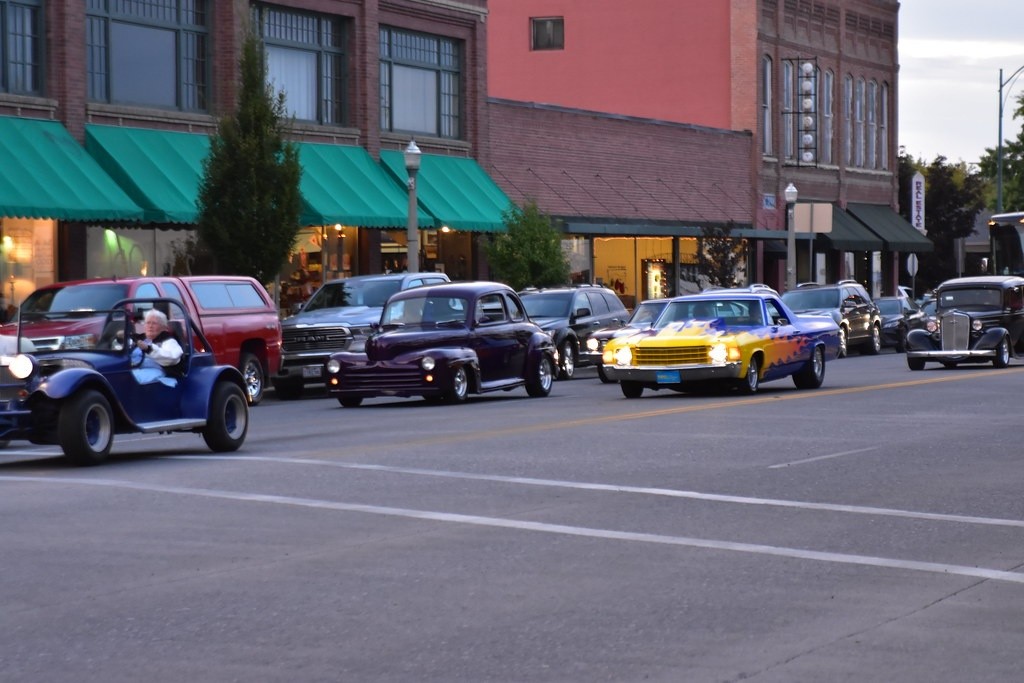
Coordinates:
846 202 934 252
275 142 436 231
0 116 142 222
379 150 526 232
86 125 226 231
787 201 883 253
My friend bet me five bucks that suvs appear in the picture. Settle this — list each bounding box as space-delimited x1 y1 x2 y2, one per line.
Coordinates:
781 278 882 359
511 283 631 380
687 283 784 318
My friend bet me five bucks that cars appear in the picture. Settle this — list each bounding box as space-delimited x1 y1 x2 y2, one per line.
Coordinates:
584 294 750 384
901 274 1024 370
872 295 921 354
322 280 561 409
920 299 952 316
1 297 251 466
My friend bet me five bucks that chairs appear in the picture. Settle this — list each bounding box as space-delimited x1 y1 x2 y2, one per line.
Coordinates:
96 320 135 352
167 321 187 361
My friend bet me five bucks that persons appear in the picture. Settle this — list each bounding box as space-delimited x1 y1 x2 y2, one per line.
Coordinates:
742 302 782 326
116 310 184 383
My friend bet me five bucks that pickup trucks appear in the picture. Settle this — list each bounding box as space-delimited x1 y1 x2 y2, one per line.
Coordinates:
0 273 282 408
270 271 502 400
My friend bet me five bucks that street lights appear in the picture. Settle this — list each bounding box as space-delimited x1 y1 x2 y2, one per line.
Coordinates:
784 182 800 291
402 140 424 274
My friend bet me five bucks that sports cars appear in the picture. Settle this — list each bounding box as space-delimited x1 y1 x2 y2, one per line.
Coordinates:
602 291 843 398
